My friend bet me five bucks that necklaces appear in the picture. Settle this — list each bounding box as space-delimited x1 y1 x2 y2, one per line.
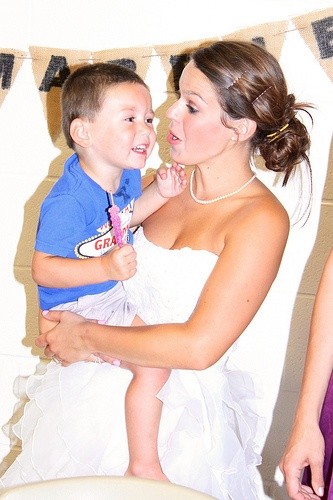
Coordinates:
189 169 257 204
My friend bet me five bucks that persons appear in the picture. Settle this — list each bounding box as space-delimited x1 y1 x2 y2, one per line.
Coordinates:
0 39 333 500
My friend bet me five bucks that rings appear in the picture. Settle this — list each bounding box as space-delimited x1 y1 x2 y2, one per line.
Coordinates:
52 356 60 365
